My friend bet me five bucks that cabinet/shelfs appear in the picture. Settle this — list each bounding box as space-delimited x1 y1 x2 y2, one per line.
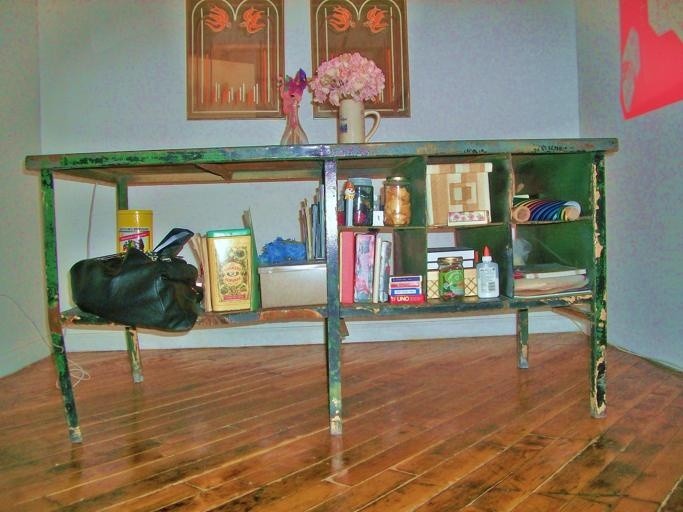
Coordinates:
25 137 619 444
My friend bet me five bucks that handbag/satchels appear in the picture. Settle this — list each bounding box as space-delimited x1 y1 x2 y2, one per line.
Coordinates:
71 227 205 333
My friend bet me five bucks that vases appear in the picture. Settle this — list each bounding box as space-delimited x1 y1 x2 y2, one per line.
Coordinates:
280 104 309 146
338 100 380 143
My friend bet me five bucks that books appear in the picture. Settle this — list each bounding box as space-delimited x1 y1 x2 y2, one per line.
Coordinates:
515 262 586 279
427 227 474 271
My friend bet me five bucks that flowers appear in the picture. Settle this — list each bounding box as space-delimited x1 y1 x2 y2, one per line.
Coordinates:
277 69 308 128
308 52 385 106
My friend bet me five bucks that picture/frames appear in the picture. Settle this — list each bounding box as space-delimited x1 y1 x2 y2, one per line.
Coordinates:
310 0 411 118
185 0 284 119
